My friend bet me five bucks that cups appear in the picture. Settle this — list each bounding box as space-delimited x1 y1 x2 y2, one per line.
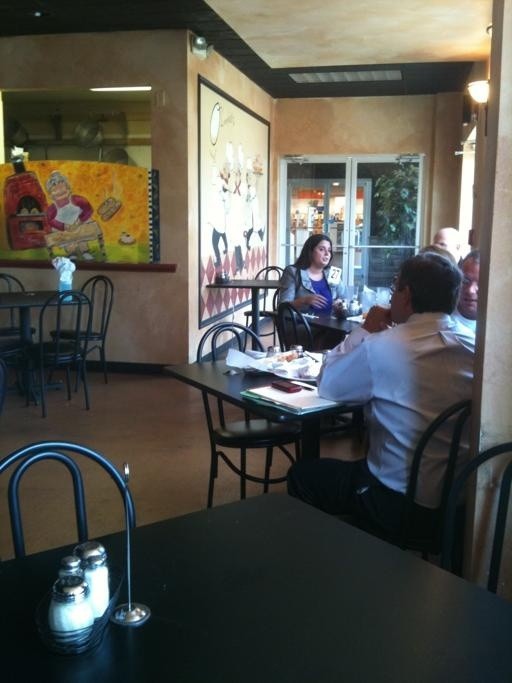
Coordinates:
58 278 73 302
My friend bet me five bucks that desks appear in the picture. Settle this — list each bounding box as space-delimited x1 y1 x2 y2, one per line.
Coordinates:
0 490 511 682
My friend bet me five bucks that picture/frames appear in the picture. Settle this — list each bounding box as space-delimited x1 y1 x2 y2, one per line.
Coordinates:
198 72 271 329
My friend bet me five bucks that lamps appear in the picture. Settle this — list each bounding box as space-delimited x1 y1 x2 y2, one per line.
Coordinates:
462 78 489 103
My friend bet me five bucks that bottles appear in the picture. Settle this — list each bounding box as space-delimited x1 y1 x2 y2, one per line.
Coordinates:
58 555 82 581
321 348 330 361
290 344 304 359
266 346 280 358
75 542 110 617
330 294 359 320
52 576 93 642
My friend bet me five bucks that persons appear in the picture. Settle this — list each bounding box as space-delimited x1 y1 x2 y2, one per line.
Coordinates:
277 234 347 352
451 250 481 334
45 172 94 261
209 142 265 275
433 227 463 258
287 251 476 552
420 245 456 265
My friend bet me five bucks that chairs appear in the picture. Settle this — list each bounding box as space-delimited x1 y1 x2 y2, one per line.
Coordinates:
332 399 511 594
0 269 116 419
0 440 138 560
164 266 364 509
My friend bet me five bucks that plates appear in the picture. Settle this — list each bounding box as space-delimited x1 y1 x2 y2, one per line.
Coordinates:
275 373 318 385
